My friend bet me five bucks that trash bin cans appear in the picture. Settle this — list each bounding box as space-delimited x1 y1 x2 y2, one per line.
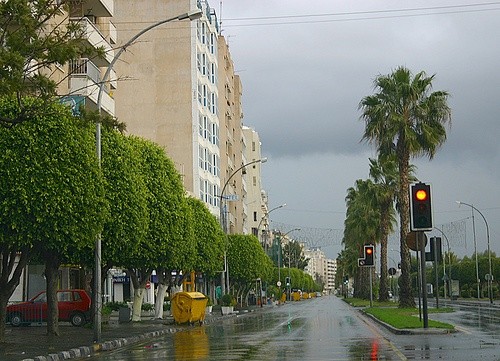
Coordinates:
169 291 207 327
281 293 286 302
302 292 308 299
291 292 300 301
310 292 316 298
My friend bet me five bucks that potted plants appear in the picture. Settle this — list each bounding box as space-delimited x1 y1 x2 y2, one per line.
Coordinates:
218 295 237 315
141 303 155 317
206 296 213 313
103 301 132 317
163 303 171 316
102 307 112 323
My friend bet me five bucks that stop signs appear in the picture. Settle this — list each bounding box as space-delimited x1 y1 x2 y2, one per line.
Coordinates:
146 282 151 289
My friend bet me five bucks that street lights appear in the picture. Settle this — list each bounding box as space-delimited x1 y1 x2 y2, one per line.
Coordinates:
219 156 269 297
456 200 494 304
278 227 302 306
93 9 205 342
289 240 308 301
256 204 287 305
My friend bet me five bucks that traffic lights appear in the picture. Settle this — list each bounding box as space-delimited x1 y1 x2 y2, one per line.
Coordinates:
357 246 376 268
409 181 433 231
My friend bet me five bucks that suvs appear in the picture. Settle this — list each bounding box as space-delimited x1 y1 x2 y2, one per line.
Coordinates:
6 289 92 327
